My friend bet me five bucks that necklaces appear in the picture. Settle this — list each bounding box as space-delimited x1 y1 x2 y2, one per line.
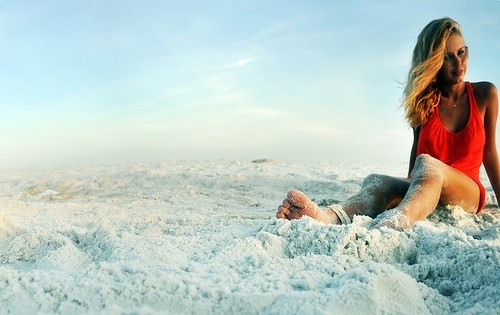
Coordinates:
438 82 465 114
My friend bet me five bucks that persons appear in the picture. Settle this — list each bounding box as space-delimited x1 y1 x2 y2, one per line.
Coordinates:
275 17 500 231
